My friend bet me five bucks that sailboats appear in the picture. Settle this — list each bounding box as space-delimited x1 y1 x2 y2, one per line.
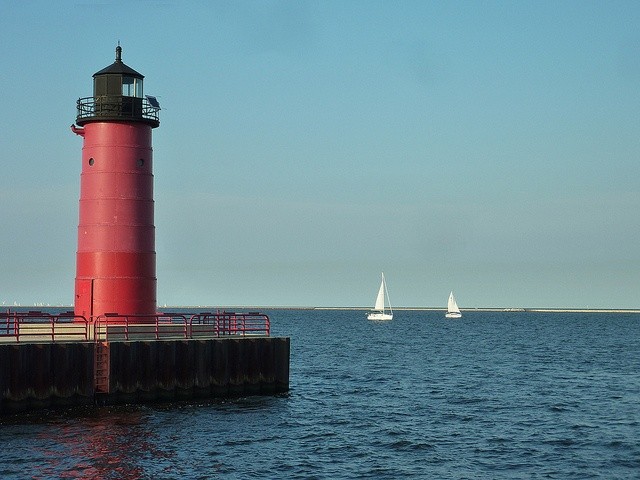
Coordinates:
366 270 394 321
446 291 462 319
